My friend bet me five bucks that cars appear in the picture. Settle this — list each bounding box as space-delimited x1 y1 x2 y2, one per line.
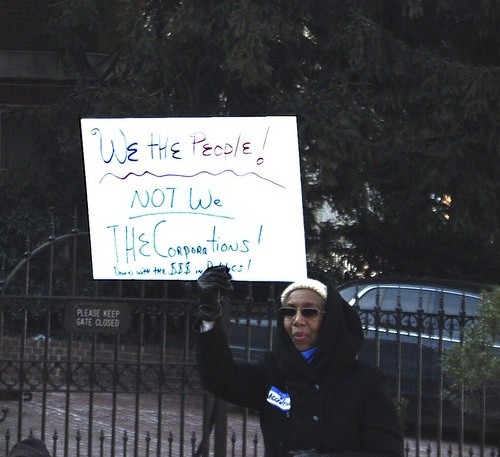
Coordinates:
227 276 500 437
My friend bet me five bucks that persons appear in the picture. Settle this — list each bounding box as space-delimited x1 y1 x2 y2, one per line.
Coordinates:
192 265 404 457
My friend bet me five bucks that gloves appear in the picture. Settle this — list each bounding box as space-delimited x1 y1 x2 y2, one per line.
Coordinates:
197 266 234 322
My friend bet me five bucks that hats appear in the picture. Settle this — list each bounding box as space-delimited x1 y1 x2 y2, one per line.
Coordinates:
280 278 328 306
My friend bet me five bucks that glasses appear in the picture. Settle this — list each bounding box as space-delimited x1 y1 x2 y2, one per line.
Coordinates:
278 307 326 318
